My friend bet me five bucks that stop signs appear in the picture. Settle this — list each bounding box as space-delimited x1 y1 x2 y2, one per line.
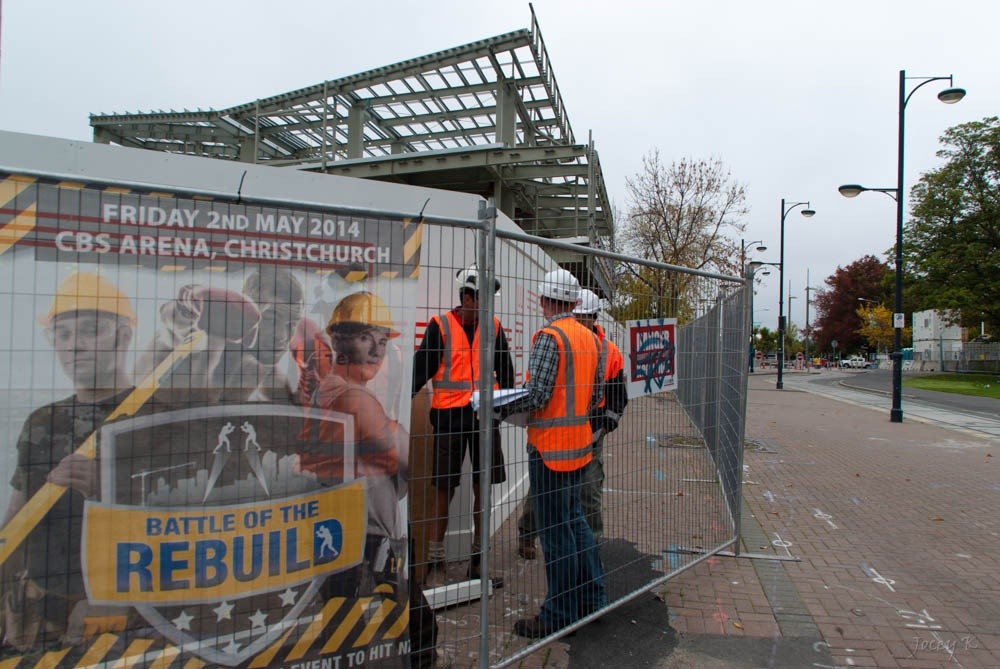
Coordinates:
797 354 803 360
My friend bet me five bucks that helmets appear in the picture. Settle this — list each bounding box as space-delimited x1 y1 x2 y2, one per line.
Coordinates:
37 271 139 324
455 264 501 297
572 288 602 313
539 269 580 302
327 290 400 339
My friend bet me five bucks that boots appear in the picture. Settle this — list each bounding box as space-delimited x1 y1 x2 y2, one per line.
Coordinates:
425 555 447 588
466 552 503 587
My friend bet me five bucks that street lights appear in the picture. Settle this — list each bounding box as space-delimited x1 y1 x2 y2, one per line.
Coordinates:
838 68 967 423
748 198 815 390
740 238 770 373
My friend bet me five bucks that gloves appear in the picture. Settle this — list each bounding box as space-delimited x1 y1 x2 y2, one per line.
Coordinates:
288 318 333 409
195 289 261 388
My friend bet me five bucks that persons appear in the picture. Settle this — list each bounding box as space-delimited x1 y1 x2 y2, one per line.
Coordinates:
411 270 630 636
1 263 412 668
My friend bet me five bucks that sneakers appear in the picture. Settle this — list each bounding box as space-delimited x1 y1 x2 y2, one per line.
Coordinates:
579 605 606 622
519 528 536 558
515 615 578 637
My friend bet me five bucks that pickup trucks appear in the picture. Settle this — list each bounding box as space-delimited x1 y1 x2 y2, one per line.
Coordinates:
841 357 872 369
